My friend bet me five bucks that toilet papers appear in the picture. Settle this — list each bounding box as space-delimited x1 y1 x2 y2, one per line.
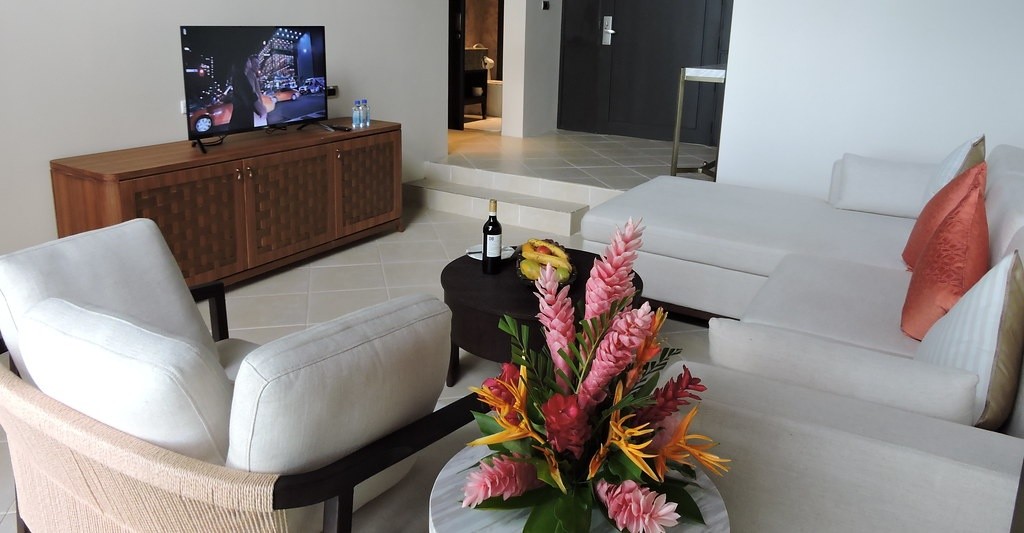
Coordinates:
482 57 494 69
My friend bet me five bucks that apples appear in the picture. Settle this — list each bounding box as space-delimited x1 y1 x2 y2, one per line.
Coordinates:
535 246 552 255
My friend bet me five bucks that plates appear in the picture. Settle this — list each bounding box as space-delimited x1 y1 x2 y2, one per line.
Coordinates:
466 244 515 260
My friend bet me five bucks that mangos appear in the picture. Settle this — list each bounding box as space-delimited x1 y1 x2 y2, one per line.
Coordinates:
555 267 570 282
520 259 543 280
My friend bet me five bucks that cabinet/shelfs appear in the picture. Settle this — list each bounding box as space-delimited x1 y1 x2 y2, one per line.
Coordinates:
464 50 488 69
50 117 405 287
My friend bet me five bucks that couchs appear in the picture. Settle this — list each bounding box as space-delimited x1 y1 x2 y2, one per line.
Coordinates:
576 145 1023 533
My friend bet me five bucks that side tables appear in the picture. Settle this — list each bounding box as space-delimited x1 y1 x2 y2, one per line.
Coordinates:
429 439 732 532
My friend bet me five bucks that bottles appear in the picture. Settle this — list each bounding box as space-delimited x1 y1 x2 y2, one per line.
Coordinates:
361 100 369 127
352 101 363 130
482 199 502 275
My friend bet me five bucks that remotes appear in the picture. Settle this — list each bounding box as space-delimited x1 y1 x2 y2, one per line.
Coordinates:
329 125 352 131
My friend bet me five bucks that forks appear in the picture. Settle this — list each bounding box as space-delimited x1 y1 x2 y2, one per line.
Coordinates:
466 247 513 254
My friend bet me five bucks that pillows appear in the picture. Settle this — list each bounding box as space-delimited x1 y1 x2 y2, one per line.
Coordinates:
900 189 992 341
19 295 233 467
914 250 1023 431
225 292 452 514
902 163 986 271
920 135 985 215
0 218 224 390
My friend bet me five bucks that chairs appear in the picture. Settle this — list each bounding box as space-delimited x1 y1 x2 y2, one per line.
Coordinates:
464 69 488 119
0 282 493 533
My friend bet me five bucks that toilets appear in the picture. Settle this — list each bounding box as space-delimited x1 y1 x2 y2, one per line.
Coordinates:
464 43 503 117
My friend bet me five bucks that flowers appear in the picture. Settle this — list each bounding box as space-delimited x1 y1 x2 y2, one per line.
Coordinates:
456 216 733 532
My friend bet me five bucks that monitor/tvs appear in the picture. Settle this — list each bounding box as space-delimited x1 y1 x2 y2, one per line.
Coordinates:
180 25 335 153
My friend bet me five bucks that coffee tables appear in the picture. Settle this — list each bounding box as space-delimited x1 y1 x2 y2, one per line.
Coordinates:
440 246 644 387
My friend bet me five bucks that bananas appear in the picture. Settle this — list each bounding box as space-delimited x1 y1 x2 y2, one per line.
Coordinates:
527 238 568 260
521 250 572 273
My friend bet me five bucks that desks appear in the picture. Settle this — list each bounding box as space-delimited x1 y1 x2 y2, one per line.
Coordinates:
670 63 728 183
